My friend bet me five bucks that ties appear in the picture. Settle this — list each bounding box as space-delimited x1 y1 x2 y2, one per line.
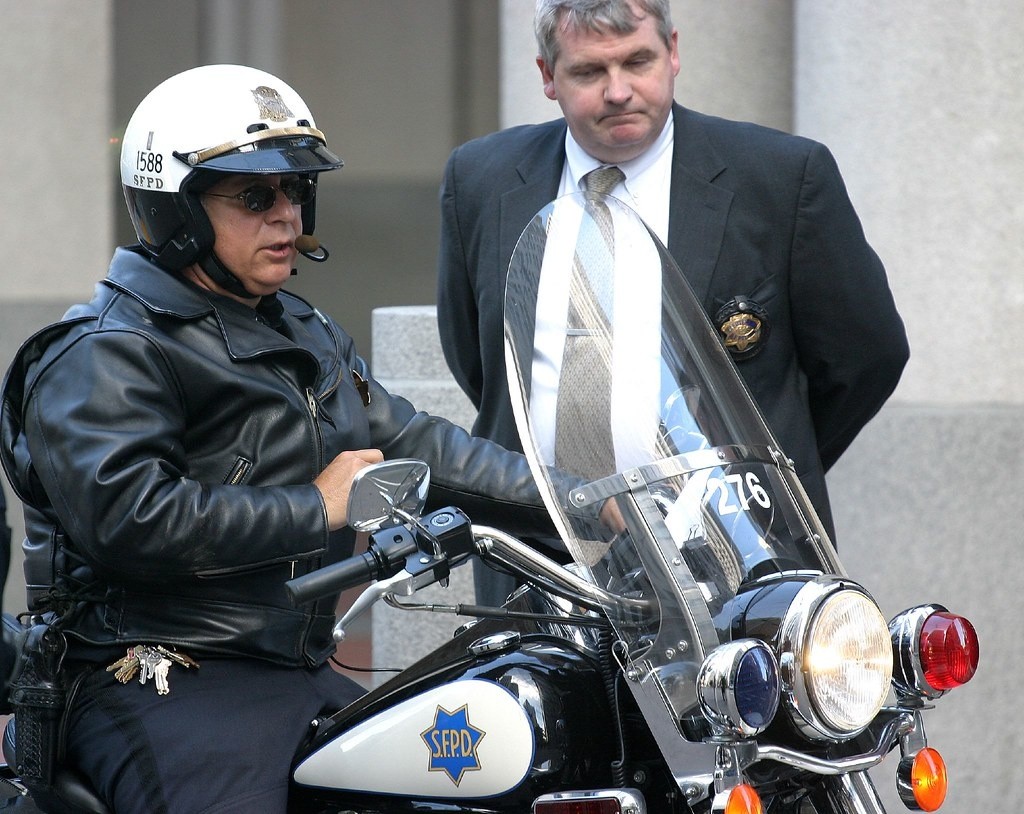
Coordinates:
555 167 628 567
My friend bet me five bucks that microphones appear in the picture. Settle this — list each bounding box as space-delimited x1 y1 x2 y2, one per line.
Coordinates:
295 234 329 262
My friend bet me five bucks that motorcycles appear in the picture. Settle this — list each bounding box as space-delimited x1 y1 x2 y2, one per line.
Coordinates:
0 187 981 814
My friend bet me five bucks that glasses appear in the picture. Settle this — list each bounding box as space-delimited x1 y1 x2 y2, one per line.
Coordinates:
203 178 319 213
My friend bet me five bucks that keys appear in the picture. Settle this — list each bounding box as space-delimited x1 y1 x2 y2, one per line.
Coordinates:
106 643 200 695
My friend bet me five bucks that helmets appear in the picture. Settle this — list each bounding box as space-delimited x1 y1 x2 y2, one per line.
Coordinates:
119 63 345 275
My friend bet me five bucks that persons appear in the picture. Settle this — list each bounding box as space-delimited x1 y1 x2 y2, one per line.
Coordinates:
0 63 628 814
437 0 911 626
